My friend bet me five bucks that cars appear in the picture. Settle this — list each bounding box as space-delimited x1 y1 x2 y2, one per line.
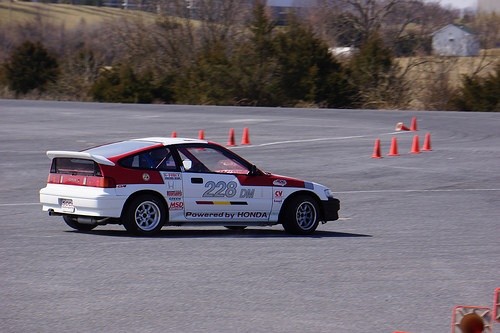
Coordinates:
38 136 341 238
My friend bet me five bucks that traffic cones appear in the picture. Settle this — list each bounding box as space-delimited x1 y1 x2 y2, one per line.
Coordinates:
421 133 433 151
386 137 400 156
408 135 423 154
240 127 252 145
451 305 495 333
226 128 238 147
198 130 205 139
370 139 384 159
394 122 410 132
171 131 177 138
409 117 419 131
